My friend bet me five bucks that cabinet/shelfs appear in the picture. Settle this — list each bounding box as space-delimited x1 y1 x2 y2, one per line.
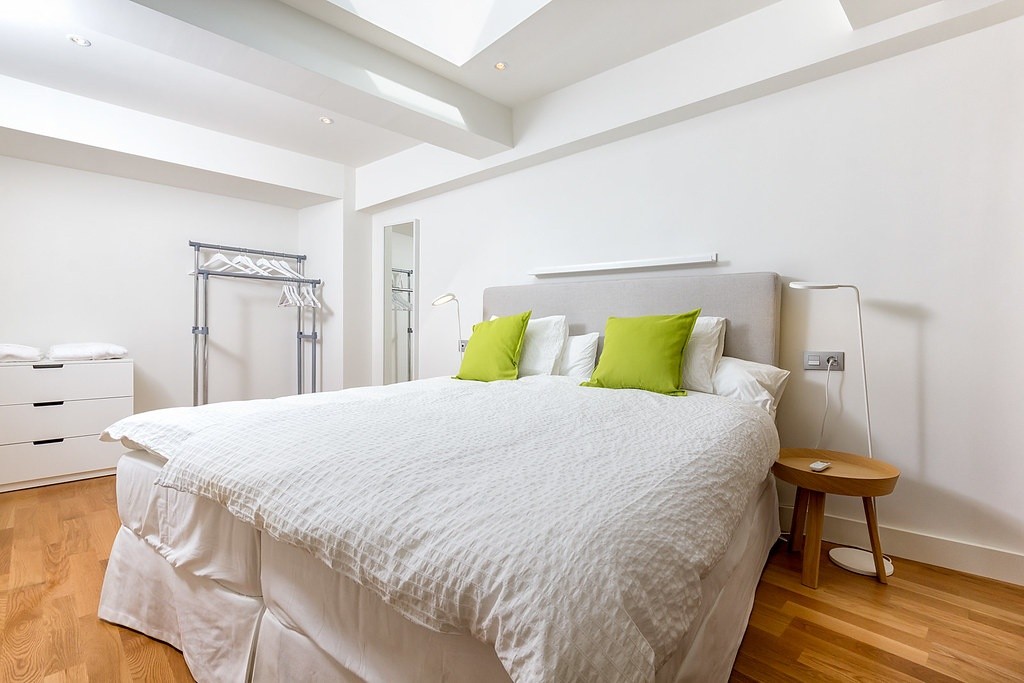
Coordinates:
0 357 135 492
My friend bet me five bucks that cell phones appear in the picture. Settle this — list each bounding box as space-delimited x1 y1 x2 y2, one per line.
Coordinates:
810 460 831 471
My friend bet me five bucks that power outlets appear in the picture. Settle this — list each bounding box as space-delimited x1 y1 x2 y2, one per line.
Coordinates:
804 351 844 371
458 340 468 351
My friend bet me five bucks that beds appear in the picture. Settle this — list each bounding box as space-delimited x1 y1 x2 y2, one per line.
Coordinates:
96 271 782 683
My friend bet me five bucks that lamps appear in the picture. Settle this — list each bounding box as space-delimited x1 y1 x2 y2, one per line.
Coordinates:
789 281 895 576
432 293 462 364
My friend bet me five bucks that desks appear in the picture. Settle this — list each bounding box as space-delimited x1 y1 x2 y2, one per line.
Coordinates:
770 448 901 589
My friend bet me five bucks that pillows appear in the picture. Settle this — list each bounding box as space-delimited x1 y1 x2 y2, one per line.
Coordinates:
681 317 725 394
559 332 598 378
488 314 569 376
713 356 790 415
578 306 703 396
449 311 532 383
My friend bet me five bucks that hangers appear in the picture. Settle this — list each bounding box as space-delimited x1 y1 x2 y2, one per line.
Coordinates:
391 288 413 310
188 245 305 279
277 277 321 308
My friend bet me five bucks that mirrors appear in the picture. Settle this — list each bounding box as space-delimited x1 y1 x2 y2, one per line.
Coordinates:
383 218 419 385
